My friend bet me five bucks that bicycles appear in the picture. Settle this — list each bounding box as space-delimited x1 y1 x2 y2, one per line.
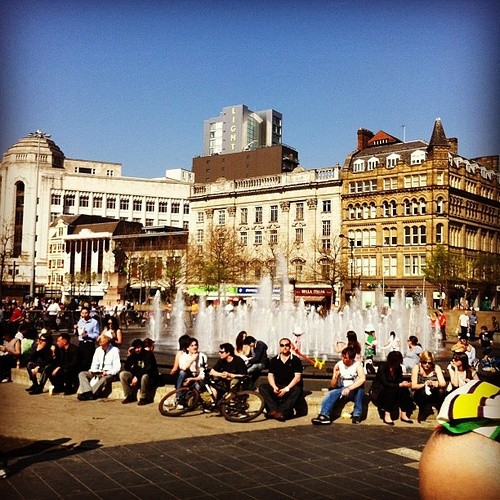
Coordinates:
159 356 265 423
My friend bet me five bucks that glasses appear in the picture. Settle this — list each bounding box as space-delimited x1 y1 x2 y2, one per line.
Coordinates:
220 351 225 354
280 344 290 347
422 360 433 365
451 357 461 362
39 337 46 342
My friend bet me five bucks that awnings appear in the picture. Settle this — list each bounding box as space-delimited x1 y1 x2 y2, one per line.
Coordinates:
272 294 280 301
294 295 325 301
206 297 218 300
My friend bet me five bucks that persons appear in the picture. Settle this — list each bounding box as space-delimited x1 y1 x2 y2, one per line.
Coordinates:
311 347 366 425
0 296 99 340
306 304 323 316
0 326 29 384
77 331 121 400
191 301 198 323
335 331 423 373
259 338 303 422
432 311 500 371
444 353 479 394
119 340 159 405
176 337 207 408
25 334 53 395
166 335 192 385
368 351 413 424
205 343 246 411
46 333 83 395
411 351 446 423
234 331 268 390
101 306 127 348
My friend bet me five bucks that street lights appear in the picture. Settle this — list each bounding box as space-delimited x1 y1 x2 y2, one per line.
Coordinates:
28 131 52 307
338 234 355 297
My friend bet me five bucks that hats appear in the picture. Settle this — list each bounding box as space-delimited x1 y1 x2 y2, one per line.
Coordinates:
293 327 305 335
459 334 469 340
364 323 375 333
132 338 155 349
179 335 190 350
451 343 467 353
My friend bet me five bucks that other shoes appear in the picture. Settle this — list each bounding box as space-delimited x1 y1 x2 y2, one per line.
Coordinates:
383 415 394 425
401 417 413 423
264 410 285 422
352 416 361 423
2 377 9 382
122 396 131 403
53 385 65 393
175 401 184 409
64 383 73 394
311 414 331 424
77 391 94 400
25 381 44 395
138 398 145 405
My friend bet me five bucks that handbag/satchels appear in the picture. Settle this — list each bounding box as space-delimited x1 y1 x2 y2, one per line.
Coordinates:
154 384 177 405
